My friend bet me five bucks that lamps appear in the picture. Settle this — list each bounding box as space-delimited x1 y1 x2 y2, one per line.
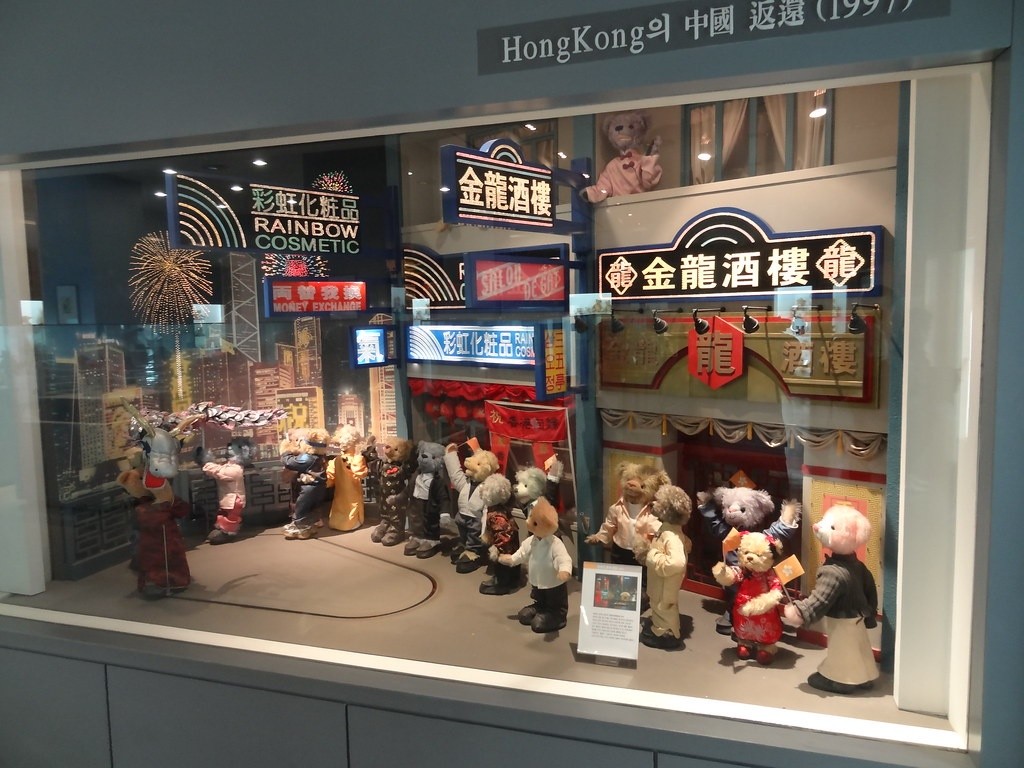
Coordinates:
693 306 726 335
790 305 822 334
742 305 773 333
848 304 876 335
651 307 684 334
574 314 590 334
610 307 646 331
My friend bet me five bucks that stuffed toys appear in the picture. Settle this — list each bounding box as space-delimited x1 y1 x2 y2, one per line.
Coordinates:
113 424 881 697
576 108 663 204
